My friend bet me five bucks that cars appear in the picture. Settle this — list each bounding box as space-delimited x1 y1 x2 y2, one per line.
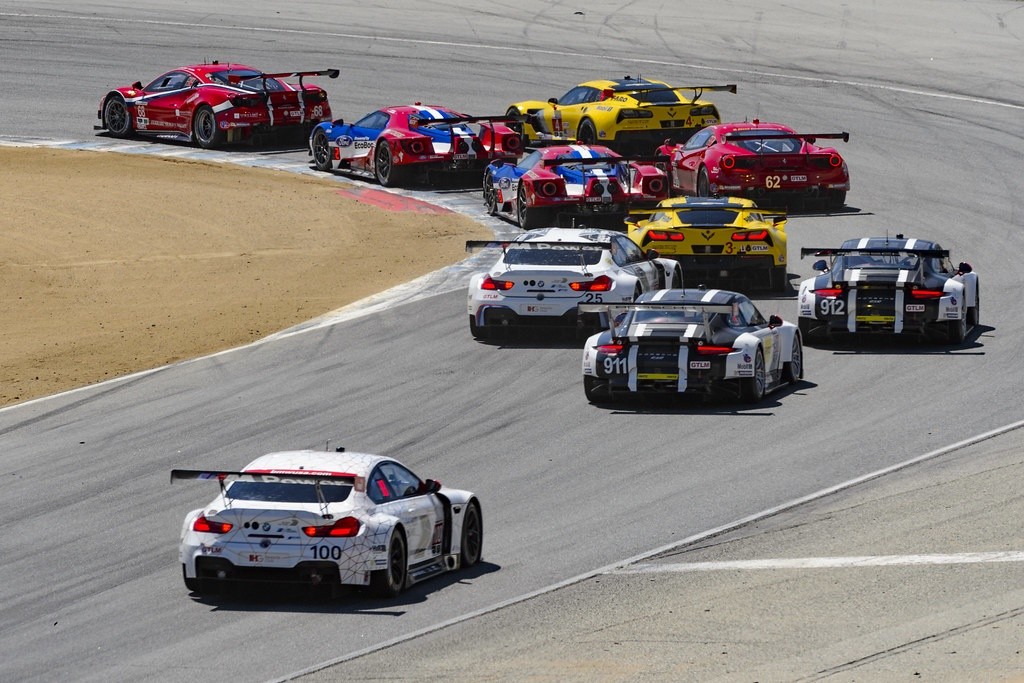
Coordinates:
483 140 670 225
307 106 531 185
464 225 684 339
95 61 340 151
507 76 738 149
655 120 853 208
581 284 805 405
795 235 983 344
167 450 484 606
623 197 790 282
